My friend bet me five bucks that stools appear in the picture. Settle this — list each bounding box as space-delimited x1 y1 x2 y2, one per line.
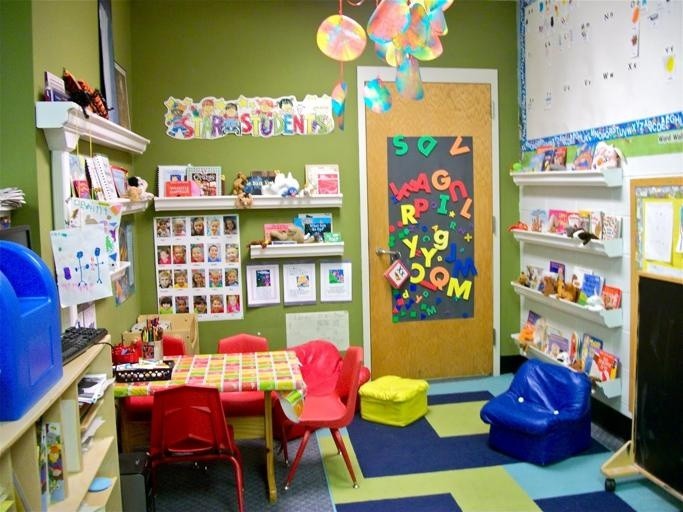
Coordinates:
146 385 244 512
283 346 364 491
358 376 430 427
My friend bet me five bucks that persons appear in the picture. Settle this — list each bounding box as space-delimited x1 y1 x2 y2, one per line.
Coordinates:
173 246 185 264
207 219 220 236
192 273 205 287
159 271 172 289
194 297 207 314
191 217 204 236
209 271 222 288
174 271 188 288
226 247 239 262
159 250 171 264
226 270 238 286
208 245 221 262
224 219 237 234
157 220 169 237
175 297 189 313
211 295 224 313
227 295 240 313
160 297 173 314
191 246 204 262
172 218 186 236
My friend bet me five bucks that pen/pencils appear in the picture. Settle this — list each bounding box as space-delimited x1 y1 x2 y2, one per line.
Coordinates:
141 315 163 342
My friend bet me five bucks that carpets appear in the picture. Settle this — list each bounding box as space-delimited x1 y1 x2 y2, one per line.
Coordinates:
314 373 683 512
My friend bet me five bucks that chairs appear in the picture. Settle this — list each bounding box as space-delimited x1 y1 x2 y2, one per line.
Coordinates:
218 334 268 356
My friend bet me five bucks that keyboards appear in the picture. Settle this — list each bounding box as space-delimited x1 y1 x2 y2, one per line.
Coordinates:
62 326 108 366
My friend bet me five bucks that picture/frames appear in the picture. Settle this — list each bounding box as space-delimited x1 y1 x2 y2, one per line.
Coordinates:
113 60 132 131
98 0 119 128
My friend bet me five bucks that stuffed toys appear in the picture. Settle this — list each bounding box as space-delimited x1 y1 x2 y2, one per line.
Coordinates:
591 141 613 170
600 147 627 171
233 171 253 209
556 280 577 302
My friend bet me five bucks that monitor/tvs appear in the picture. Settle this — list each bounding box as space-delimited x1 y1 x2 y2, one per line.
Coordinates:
0 225 32 250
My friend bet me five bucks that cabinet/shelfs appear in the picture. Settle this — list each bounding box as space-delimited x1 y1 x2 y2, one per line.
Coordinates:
510 168 629 414
36 102 154 283
0 335 123 512
154 195 344 259
122 313 199 357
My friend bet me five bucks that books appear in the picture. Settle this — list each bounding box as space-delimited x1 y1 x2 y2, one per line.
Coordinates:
298 213 333 234
548 209 622 241
318 173 338 195
0 186 27 208
85 155 118 200
164 182 192 197
156 165 187 198
305 164 340 194
293 217 331 241
545 260 566 281
264 223 294 243
77 374 117 453
577 333 602 373
36 421 65 512
71 156 91 198
602 285 621 309
526 310 580 365
12 472 32 512
0 488 15 512
581 273 605 298
110 165 129 198
585 347 620 384
187 164 222 196
522 143 594 172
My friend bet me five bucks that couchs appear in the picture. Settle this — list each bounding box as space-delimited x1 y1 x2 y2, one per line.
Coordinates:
272 340 371 442
480 358 592 466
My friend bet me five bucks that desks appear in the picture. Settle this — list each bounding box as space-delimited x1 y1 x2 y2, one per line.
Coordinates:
114 351 303 505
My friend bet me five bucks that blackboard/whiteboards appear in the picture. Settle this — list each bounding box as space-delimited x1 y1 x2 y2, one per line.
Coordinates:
631 269 682 503
